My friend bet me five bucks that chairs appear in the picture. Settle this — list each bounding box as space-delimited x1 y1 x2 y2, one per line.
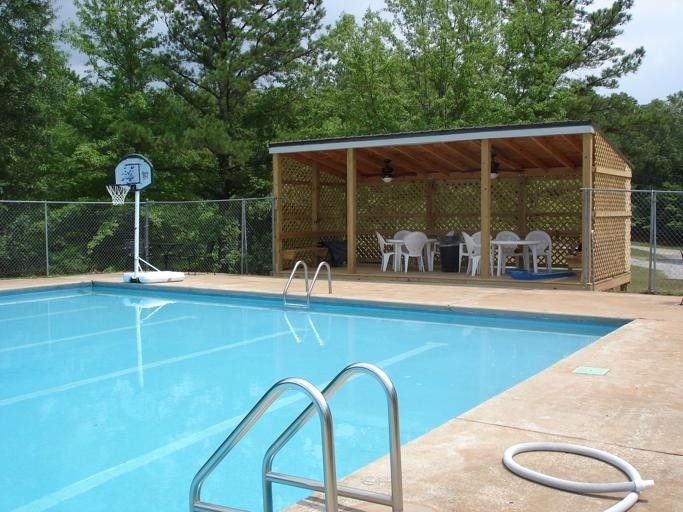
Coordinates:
374 229 455 273
458 231 551 278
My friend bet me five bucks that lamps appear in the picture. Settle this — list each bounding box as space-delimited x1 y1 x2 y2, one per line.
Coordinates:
490 151 500 179
380 159 394 183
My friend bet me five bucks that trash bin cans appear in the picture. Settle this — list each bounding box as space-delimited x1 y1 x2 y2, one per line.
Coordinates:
440 242 459 272
